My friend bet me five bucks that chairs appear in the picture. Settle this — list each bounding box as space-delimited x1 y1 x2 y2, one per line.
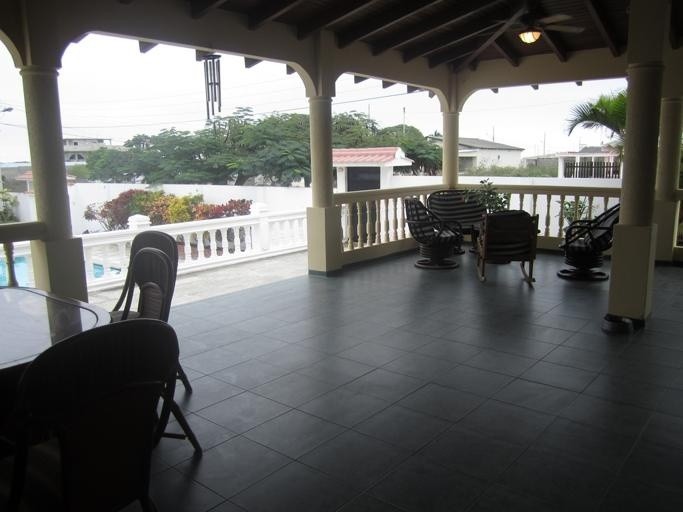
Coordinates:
120 248 201 454
406 198 464 270
474 211 540 285
0 318 179 510
110 231 194 396
556 202 621 282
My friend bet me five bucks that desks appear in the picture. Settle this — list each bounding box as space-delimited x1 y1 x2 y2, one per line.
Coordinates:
1 287 115 372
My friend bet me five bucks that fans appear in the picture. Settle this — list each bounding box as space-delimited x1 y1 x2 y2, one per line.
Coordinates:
476 1 588 43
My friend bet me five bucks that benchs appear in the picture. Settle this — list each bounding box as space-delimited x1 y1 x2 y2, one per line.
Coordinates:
427 190 492 253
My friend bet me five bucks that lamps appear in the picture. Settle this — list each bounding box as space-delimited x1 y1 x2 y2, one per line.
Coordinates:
516 13 541 43
